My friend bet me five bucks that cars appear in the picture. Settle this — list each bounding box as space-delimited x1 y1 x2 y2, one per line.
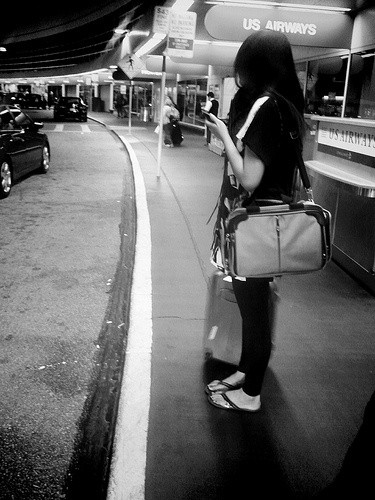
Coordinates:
53 96 89 122
0 104 51 200
0 90 52 111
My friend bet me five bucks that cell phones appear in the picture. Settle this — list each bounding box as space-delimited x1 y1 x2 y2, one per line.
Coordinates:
201 109 228 128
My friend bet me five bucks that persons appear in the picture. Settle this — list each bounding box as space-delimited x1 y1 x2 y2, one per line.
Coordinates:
162 96 181 148
202 30 307 412
200 92 219 148
116 92 125 119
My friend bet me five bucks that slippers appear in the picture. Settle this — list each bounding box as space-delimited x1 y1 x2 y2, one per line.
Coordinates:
204 378 234 394
208 391 260 412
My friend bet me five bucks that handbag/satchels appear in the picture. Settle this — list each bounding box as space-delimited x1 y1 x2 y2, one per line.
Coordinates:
223 198 332 278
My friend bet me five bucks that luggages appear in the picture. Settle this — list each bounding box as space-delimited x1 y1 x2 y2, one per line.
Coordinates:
170 121 183 147
